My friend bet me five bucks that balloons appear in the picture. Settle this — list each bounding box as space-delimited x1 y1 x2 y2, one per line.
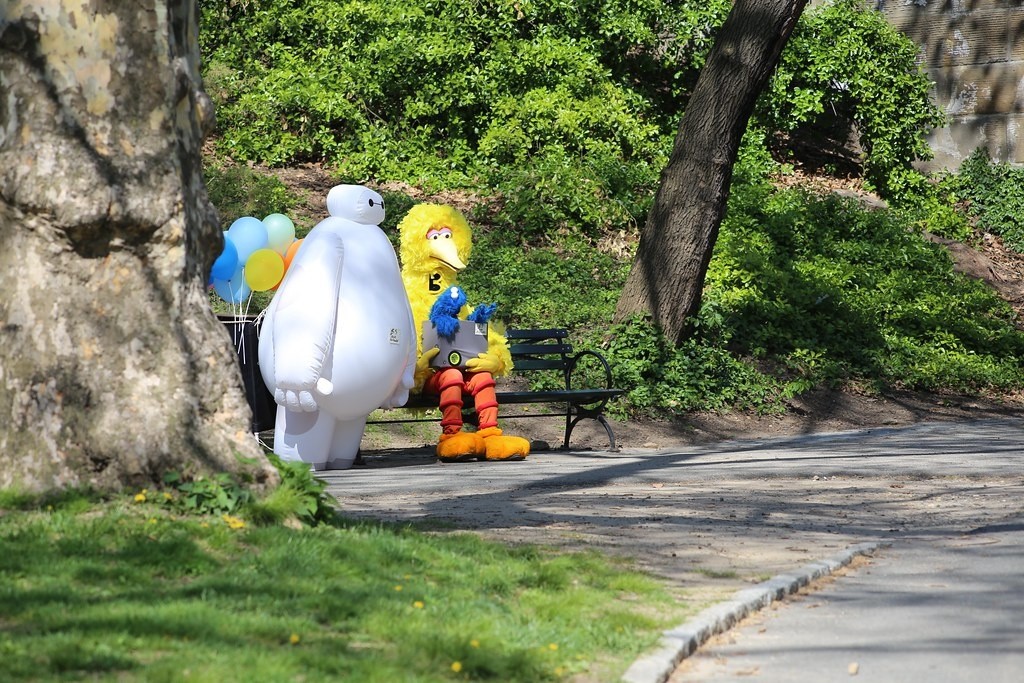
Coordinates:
211 237 237 283
271 254 288 290
208 231 249 303
245 248 283 291
263 214 295 254
228 217 268 267
286 239 305 265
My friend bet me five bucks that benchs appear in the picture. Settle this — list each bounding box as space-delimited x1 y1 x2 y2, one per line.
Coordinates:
367 330 627 453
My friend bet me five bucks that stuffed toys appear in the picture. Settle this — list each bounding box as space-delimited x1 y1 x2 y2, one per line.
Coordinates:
399 202 529 461
